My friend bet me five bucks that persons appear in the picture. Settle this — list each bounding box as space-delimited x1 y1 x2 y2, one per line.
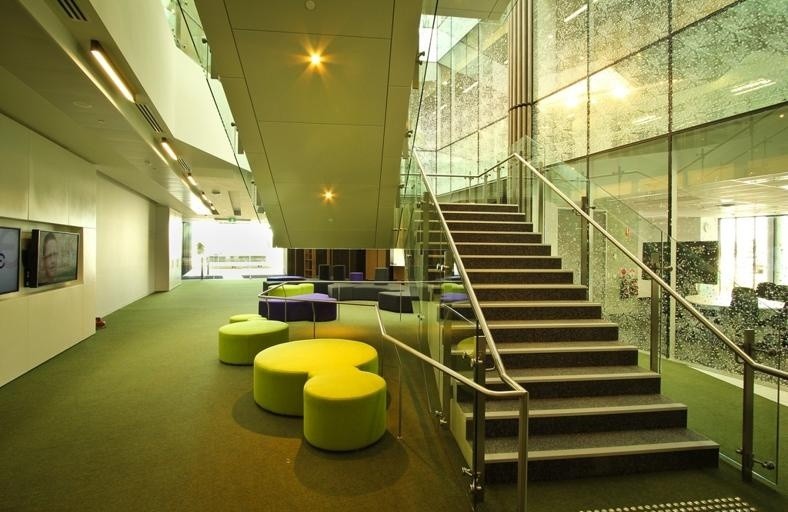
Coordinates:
43 232 58 283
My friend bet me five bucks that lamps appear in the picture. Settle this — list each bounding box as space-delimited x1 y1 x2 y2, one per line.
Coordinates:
86 38 219 215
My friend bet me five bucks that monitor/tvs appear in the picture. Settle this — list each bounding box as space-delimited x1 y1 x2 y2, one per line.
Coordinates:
32 229 80 288
0 226 20 296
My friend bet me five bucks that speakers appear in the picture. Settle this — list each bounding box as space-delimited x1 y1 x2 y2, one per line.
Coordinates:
318 264 329 280
334 265 346 281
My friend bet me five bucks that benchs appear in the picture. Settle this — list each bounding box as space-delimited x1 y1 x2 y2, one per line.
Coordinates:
219 313 290 367
255 266 470 318
255 339 388 451
